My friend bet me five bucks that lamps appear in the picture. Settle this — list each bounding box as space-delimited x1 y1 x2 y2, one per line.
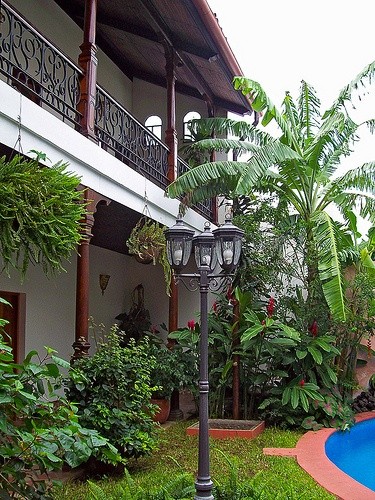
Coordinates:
207 52 220 64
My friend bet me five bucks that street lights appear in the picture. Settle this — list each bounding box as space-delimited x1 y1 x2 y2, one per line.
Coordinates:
164 213 245 500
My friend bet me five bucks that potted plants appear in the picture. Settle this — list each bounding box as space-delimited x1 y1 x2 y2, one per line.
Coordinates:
134 326 191 423
127 204 166 266
